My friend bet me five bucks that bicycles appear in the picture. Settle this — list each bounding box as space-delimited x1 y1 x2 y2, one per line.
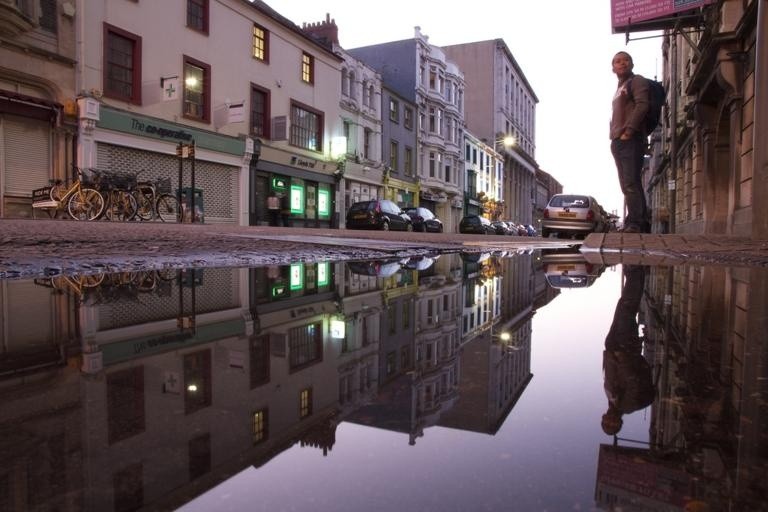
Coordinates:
35 268 178 306
30 163 184 223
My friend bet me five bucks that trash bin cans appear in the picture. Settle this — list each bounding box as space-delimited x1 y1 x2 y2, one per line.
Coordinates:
176 187 205 224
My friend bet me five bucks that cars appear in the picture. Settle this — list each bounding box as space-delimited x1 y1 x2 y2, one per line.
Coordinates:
542 252 604 288
540 196 613 239
349 257 411 279
459 216 538 237
405 253 441 271
460 248 535 262
346 199 413 233
402 207 444 233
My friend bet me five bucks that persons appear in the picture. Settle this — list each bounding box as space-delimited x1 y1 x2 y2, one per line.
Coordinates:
609 50 653 232
278 191 290 226
267 191 280 226
601 265 656 435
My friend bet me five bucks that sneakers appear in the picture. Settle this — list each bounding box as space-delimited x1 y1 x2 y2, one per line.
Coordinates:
619 223 651 233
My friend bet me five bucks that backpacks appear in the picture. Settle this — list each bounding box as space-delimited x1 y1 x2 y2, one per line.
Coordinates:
613 354 657 417
626 74 666 138
